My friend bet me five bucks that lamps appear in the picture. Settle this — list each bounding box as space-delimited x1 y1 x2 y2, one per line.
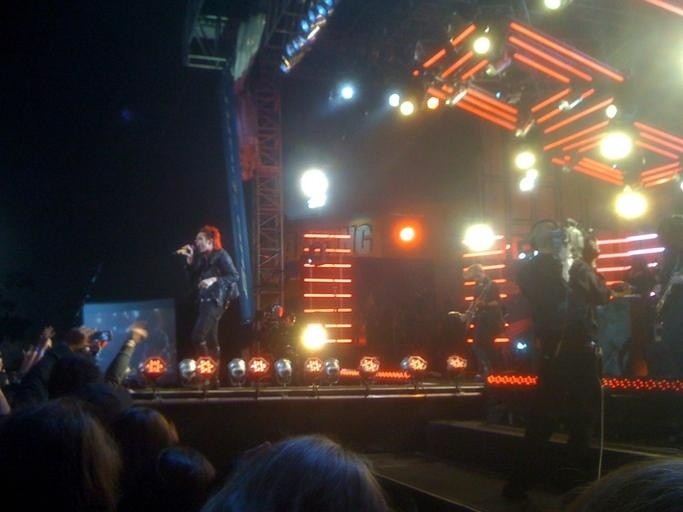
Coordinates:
279 0 335 73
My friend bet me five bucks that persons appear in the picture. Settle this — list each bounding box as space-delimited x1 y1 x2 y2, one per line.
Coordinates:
1 326 389 512
467 264 504 377
503 220 609 498
623 257 655 376
183 226 240 360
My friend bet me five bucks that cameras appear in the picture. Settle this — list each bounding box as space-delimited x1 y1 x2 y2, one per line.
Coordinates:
90 330 112 341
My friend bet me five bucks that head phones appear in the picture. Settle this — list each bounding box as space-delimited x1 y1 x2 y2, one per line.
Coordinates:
527 219 559 247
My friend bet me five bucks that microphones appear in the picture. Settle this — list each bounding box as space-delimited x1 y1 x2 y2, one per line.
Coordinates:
173 245 195 257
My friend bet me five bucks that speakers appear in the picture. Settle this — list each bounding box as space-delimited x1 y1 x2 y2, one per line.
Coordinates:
178 317 213 391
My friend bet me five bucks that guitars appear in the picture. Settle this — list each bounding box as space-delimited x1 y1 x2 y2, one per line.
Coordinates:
465 280 489 322
